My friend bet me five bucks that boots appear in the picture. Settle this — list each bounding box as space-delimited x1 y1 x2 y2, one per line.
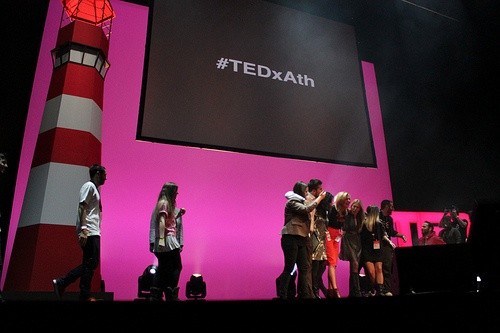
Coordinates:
350 272 361 297
149 286 180 302
377 284 384 296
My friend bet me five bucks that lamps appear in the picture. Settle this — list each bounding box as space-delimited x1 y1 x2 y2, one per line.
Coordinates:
137 264 156 298
185 274 207 299
275 270 297 297
359 273 372 296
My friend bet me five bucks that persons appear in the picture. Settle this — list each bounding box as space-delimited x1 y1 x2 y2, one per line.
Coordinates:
417 221 446 245
149 182 185 302
52 163 106 302
278 178 407 298
439 205 467 244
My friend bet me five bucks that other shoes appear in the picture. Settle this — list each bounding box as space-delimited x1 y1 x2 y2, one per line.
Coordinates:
326 289 342 299
52 277 67 299
384 292 393 296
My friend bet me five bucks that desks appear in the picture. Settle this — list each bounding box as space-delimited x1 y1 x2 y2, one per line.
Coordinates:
395 245 483 294
